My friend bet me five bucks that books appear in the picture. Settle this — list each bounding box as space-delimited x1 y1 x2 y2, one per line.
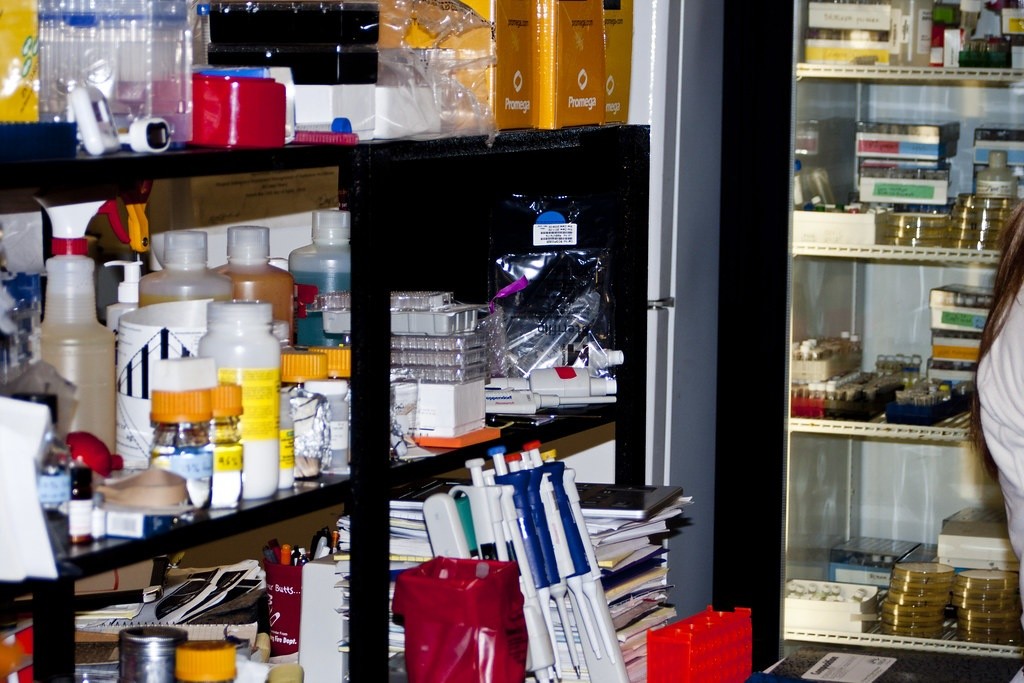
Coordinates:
334 483 683 683
830 283 1019 585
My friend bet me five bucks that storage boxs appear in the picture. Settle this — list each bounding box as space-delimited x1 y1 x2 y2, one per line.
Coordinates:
927 282 991 332
972 122 1023 166
377 0 537 132
603 0 633 124
534 0 606 129
929 328 982 363
828 537 920 589
792 203 894 245
858 159 953 206
855 115 961 161
411 380 486 438
784 578 878 633
146 165 342 235
936 520 1019 572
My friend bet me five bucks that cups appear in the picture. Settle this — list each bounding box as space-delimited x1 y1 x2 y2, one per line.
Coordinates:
260 557 311 657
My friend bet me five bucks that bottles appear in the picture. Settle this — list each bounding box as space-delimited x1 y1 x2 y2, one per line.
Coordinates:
791 331 860 402
932 292 992 395
975 150 1017 200
877 354 922 389
0 200 350 562
175 641 236 683
117 628 187 683
793 160 869 213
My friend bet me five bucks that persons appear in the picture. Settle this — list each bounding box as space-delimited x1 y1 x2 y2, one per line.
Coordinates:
973 198 1024 629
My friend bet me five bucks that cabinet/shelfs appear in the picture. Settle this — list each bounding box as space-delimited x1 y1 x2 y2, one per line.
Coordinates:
712 0 1024 667
0 124 652 683
